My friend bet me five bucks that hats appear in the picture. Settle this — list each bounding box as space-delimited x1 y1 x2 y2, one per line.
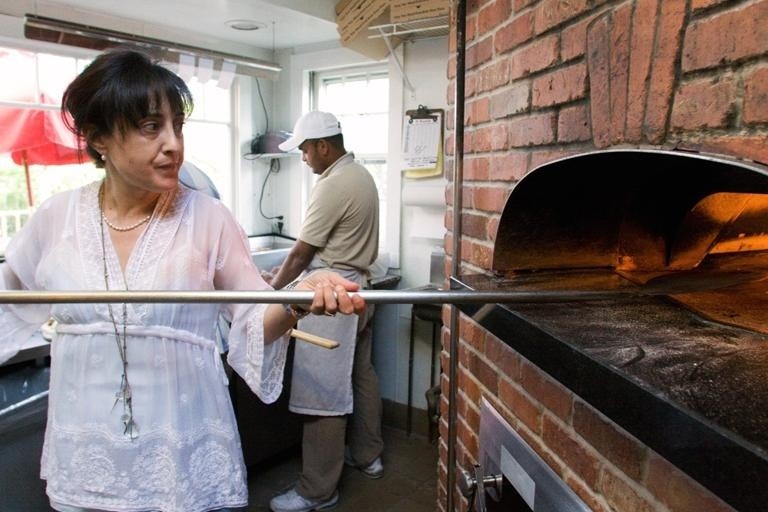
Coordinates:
278 110 342 152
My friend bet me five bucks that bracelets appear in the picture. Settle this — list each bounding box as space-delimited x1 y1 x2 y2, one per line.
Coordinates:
281 278 310 320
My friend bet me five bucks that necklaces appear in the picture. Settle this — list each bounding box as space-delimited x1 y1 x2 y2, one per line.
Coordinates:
99 171 163 438
102 210 151 231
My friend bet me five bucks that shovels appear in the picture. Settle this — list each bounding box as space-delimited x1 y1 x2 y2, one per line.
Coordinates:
2 270 767 309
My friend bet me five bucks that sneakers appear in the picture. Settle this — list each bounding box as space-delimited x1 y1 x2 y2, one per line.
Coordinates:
344 445 384 480
270 485 339 512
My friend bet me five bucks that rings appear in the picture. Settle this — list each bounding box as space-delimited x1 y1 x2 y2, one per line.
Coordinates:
324 310 337 319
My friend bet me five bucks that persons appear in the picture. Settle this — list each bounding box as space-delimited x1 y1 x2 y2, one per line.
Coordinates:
263 109 382 511
0 50 369 511
